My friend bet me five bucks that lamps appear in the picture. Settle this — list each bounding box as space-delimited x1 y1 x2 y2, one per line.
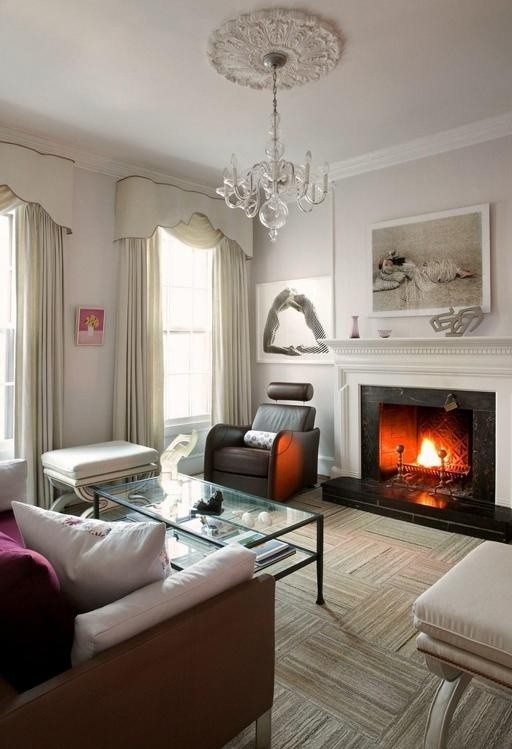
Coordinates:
207 7 345 245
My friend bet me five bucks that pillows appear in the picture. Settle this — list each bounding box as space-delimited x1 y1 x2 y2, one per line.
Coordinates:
244 430 277 450
1 500 173 645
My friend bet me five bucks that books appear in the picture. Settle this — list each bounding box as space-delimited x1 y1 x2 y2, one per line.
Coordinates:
124 493 296 578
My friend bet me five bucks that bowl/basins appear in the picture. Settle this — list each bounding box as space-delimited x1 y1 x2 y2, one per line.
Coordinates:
377 329 392 339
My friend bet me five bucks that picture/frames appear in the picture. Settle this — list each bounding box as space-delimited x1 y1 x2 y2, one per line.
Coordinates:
76 307 106 346
256 275 333 366
366 202 491 319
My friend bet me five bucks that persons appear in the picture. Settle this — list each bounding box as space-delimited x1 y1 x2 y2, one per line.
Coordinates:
295 290 330 353
262 288 307 356
378 248 475 293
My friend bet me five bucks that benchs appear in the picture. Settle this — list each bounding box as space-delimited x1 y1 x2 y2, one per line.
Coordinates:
412 539 512 749
41 439 159 520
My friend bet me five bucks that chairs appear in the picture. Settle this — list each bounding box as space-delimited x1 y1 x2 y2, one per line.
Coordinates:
203 382 320 503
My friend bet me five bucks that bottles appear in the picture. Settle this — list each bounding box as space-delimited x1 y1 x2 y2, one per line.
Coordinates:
350 314 361 338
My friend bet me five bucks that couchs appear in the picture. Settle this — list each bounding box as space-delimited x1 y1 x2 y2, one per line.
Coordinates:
0 459 274 749
